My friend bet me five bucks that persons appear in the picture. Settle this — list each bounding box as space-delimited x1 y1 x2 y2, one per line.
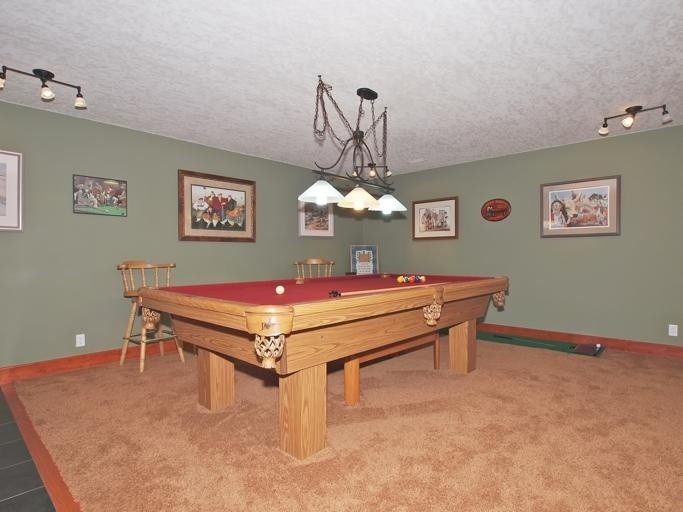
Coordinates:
75 176 126 210
191 191 245 228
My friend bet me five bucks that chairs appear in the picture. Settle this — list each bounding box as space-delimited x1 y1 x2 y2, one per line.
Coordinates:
116 260 185 372
293 258 335 278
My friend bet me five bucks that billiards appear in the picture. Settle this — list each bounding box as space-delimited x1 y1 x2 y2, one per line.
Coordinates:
276 285 284 294
396 275 426 282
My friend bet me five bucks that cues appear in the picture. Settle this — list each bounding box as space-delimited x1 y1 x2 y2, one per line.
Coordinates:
339 282 450 296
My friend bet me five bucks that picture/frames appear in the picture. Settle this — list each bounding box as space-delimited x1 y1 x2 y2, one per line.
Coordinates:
0 150 22 231
73 174 127 217
412 197 458 239
540 175 621 237
350 245 379 275
178 169 256 243
299 201 334 236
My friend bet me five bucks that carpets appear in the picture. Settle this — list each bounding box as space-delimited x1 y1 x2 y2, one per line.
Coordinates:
1 333 683 512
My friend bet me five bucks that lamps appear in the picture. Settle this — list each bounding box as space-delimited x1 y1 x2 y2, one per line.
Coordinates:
599 104 673 135
0 66 86 108
297 74 408 216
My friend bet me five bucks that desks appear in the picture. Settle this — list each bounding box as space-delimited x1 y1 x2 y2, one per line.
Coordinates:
137 272 510 461
